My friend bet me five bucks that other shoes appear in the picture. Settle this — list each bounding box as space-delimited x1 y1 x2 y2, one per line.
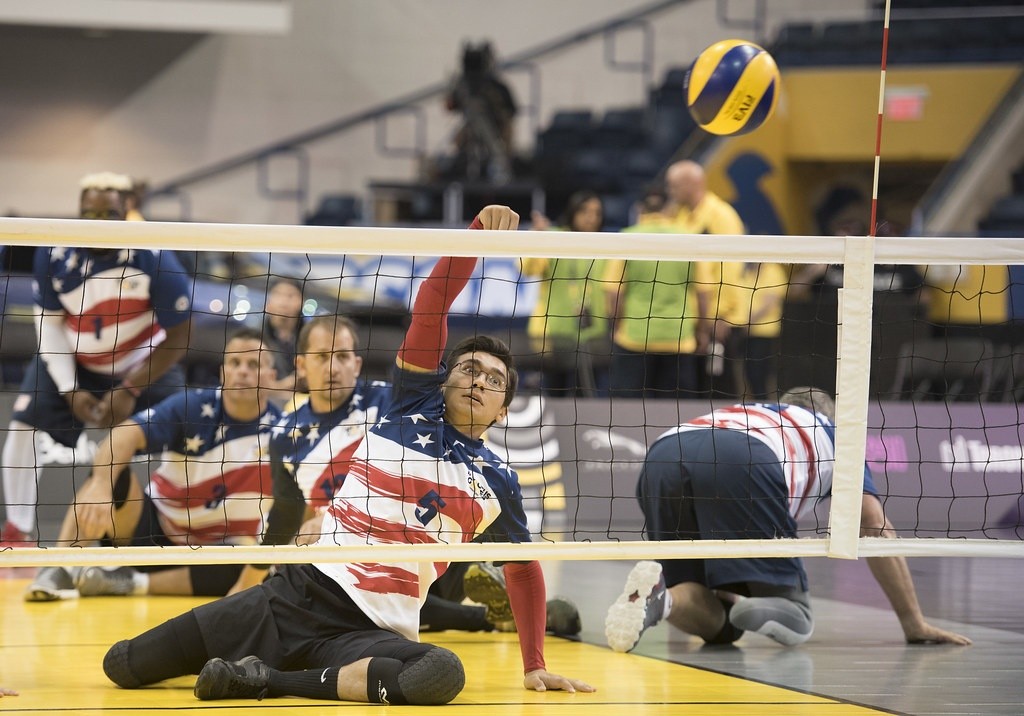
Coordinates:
463 562 515 631
1 522 36 547
543 596 581 636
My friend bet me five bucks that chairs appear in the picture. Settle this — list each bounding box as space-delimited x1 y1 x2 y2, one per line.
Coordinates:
892 340 992 405
303 16 1024 234
1000 347 1024 403
579 339 616 398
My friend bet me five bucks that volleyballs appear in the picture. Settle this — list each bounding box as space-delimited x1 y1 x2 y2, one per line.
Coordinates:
680 37 783 134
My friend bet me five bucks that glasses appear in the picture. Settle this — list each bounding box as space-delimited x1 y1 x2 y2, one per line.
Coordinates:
446 363 507 390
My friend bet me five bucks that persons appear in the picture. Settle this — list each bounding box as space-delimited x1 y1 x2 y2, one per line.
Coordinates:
602 386 972 653
2 159 786 706
102 204 598 705
443 39 518 181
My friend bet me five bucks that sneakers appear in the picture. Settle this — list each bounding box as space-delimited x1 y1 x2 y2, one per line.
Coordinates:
195 656 271 701
76 561 138 597
25 567 80 599
605 560 667 652
